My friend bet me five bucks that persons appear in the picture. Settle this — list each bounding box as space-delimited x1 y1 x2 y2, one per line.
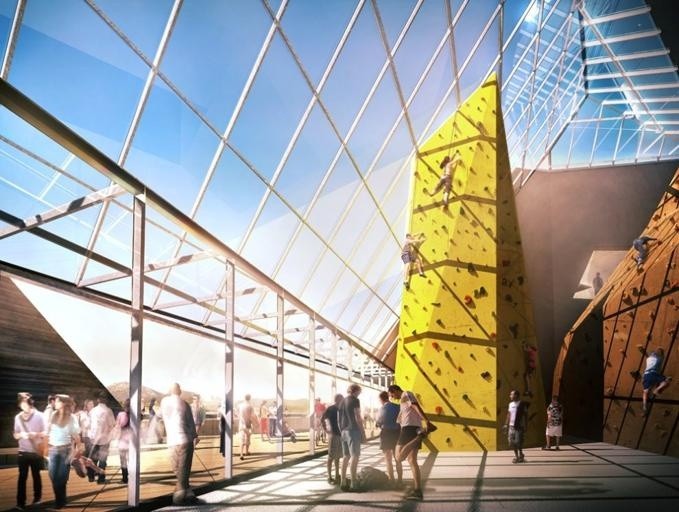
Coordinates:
311 381 437 500
640 349 667 413
542 395 565 450
522 340 537 396
401 233 426 288
503 390 529 463
592 272 603 296
632 235 657 268
12 383 277 512
423 149 461 207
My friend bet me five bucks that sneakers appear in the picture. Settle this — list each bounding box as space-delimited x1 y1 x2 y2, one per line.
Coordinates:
14 499 66 511
511 458 518 464
425 423 436 431
405 489 422 501
335 475 341 483
519 454 524 461
327 477 334 484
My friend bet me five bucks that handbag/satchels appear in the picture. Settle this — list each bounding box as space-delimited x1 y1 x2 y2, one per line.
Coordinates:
36 455 48 470
